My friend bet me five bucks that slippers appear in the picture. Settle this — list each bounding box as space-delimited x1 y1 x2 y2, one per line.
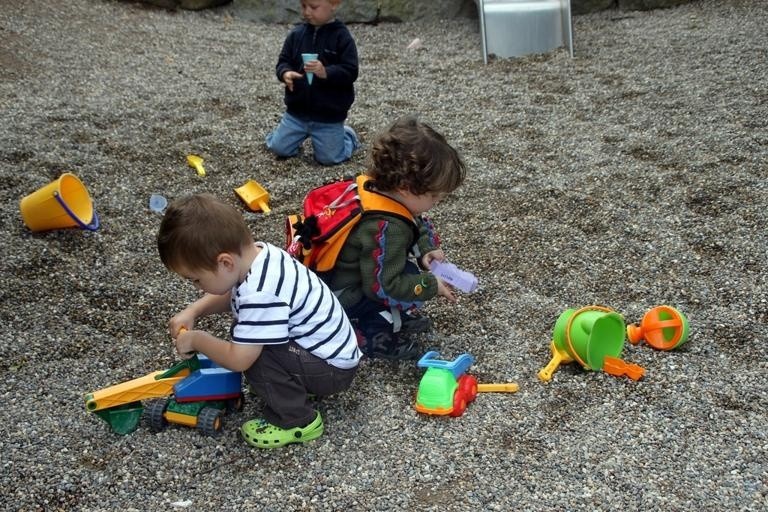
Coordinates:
241 409 324 448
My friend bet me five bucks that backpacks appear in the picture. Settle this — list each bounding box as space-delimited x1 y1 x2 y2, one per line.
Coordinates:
286 174 419 281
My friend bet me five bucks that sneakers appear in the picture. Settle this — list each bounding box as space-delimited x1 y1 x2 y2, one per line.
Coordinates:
398 311 431 332
361 326 419 359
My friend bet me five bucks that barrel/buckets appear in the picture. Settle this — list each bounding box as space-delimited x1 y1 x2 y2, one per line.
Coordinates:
20 173 99 231
553 305 626 372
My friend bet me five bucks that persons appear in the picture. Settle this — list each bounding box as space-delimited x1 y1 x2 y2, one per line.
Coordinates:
286 116 465 360
156 195 361 450
266 0 359 166
